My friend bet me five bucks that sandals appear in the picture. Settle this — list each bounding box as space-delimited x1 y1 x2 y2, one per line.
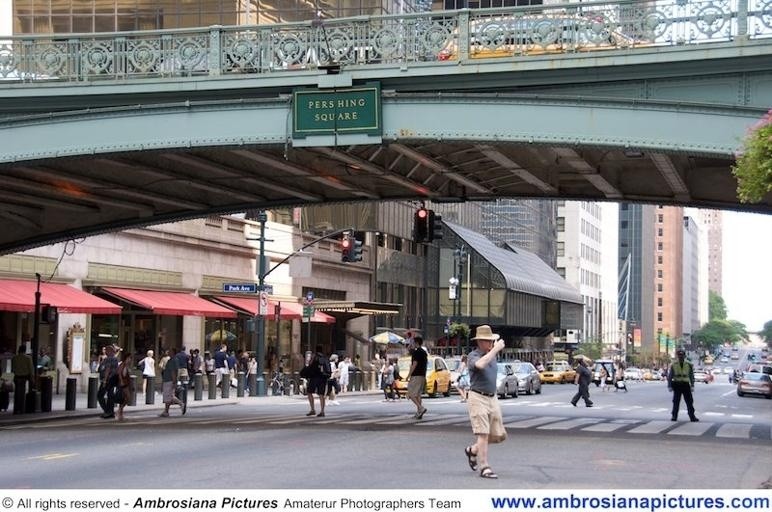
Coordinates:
464 445 478 471
481 467 497 478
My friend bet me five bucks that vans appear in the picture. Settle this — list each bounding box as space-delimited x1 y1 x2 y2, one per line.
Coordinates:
438 16 645 62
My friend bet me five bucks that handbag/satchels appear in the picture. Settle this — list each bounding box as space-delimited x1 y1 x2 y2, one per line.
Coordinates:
137 361 145 370
300 366 313 378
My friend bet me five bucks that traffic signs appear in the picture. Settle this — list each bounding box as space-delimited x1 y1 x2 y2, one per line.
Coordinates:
224 283 256 292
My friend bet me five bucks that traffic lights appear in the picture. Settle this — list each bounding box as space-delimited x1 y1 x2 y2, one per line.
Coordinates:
411 208 443 242
341 234 364 263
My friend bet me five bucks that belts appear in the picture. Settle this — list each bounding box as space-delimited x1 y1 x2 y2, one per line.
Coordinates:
471 389 495 397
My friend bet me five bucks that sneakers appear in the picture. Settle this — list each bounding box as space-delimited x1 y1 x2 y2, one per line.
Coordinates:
307 410 324 417
101 410 128 422
415 407 427 419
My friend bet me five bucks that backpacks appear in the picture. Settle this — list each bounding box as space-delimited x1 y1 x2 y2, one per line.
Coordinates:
316 356 331 379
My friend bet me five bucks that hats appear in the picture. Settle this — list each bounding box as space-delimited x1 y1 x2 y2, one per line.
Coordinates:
471 324 500 340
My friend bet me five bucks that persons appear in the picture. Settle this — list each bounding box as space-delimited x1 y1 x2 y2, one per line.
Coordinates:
268 336 279 380
635 361 669 382
453 354 471 402
668 349 699 422
568 358 628 407
37 347 52 374
406 337 428 420
370 344 404 401
535 359 546 372
407 332 415 349
704 370 714 384
728 369 740 384
95 344 259 422
465 325 507 478
306 344 365 417
11 345 35 415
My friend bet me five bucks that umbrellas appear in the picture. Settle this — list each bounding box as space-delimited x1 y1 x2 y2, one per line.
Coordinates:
367 330 408 356
205 327 237 343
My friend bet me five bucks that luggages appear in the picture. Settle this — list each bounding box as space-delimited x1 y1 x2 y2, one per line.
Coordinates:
0 387 9 412
26 384 42 413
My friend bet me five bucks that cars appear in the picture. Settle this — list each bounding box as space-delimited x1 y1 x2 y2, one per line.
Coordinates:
748 348 772 373
460 359 541 397
541 365 577 384
624 367 662 380
705 346 739 374
738 372 772 398
693 369 712 382
385 354 460 398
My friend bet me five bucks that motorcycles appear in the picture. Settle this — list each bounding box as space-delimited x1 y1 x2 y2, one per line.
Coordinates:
592 359 623 386
